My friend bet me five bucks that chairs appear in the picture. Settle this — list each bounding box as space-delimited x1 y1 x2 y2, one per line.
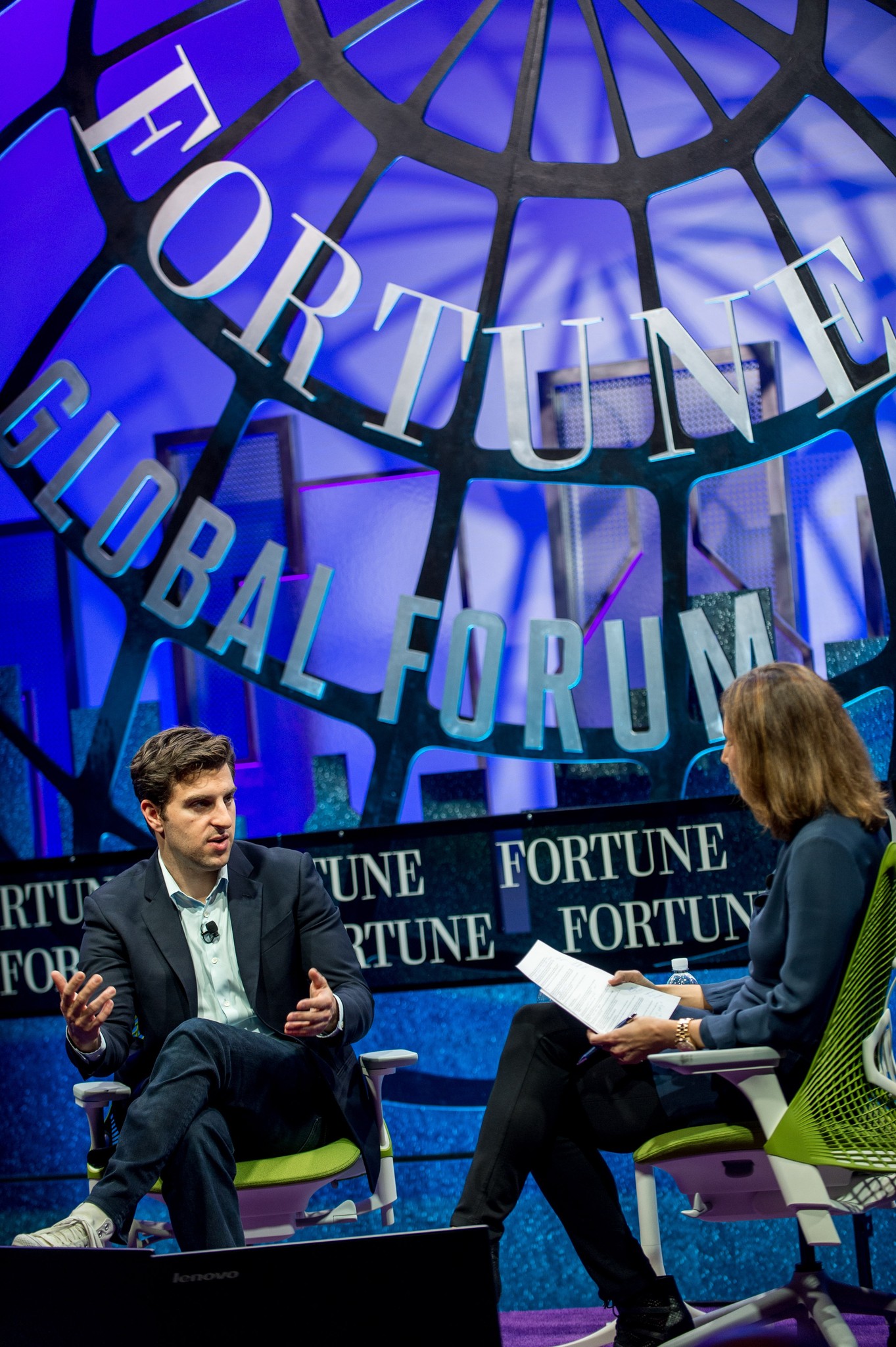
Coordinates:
62 1033 423 1248
625 847 892 1347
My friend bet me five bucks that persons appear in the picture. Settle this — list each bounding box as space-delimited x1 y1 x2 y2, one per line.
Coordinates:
11 726 382 1253
449 661 895 1347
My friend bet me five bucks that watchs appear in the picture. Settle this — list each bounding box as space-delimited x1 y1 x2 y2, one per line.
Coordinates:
674 1017 697 1052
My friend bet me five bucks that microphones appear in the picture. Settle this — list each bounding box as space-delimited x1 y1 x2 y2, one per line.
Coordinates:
200 920 219 943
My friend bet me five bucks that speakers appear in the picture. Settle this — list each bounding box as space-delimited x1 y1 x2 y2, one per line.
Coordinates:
0 1224 505 1346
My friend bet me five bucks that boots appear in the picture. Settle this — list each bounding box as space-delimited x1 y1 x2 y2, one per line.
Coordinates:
610 1274 697 1347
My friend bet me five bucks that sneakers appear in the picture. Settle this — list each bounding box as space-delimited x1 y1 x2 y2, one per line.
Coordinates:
13 1202 113 1249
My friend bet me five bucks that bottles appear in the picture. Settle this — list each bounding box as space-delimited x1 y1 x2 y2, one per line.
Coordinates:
666 958 699 985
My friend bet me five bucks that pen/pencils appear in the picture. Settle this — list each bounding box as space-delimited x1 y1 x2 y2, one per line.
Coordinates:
575 1013 637 1066
